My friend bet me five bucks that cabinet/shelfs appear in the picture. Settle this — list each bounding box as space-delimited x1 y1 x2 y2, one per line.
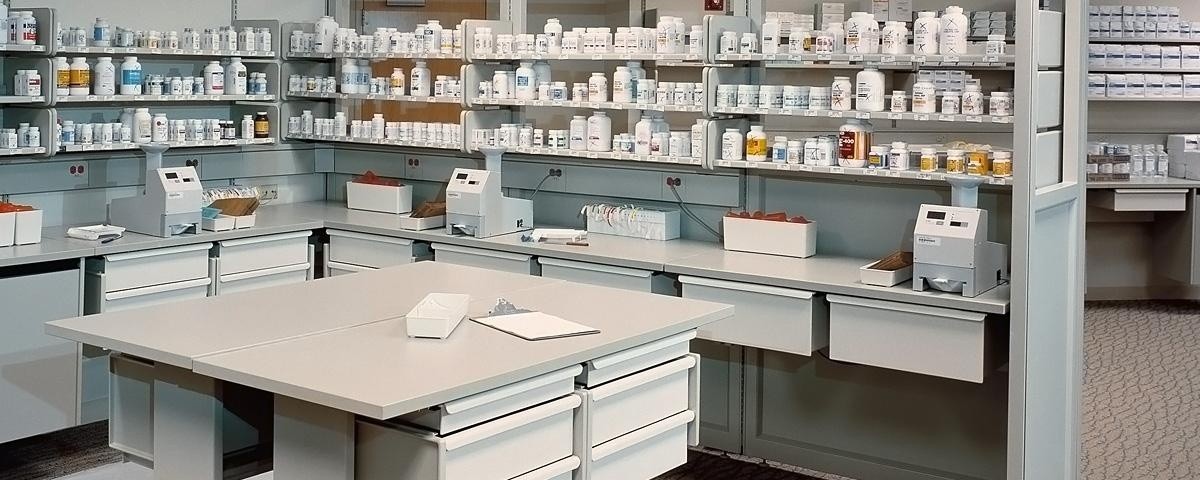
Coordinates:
0 0 324 445
282 1 1089 480
1085 37 1200 304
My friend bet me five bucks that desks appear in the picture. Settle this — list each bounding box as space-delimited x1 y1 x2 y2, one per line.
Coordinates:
46 260 737 480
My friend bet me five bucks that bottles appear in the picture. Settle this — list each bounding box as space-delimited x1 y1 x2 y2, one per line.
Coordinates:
472 16 703 157
719 118 1014 177
15 70 41 95
55 18 271 146
720 5 1014 54
1086 143 1168 182
717 65 1011 116
0 0 36 44
289 16 461 149
0 123 40 149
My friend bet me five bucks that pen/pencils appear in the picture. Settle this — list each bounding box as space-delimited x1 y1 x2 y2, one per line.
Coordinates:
101 235 123 243
